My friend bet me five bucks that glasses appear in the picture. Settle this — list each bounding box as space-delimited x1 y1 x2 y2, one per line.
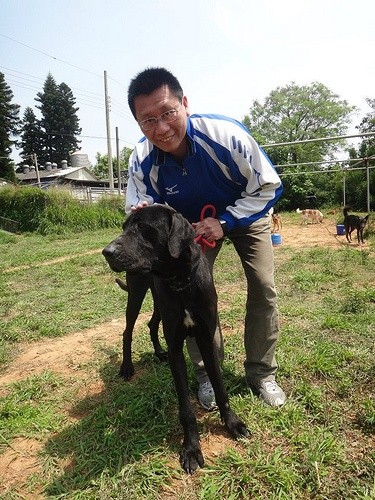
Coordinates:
138 102 182 131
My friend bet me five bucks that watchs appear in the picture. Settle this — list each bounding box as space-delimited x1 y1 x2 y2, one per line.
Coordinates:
219 219 228 236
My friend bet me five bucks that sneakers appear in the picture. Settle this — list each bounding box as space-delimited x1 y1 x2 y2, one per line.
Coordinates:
254 374 287 408
198 381 218 411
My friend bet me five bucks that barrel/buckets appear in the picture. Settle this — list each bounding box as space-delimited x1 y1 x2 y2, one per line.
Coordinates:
337 224 346 236
271 233 281 248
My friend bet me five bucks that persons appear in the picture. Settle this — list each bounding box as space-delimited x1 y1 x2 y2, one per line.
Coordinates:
124 66 287 412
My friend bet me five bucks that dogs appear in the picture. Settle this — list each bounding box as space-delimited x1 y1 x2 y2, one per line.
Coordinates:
270 214 283 230
101 202 252 476
342 207 371 245
295 207 324 225
